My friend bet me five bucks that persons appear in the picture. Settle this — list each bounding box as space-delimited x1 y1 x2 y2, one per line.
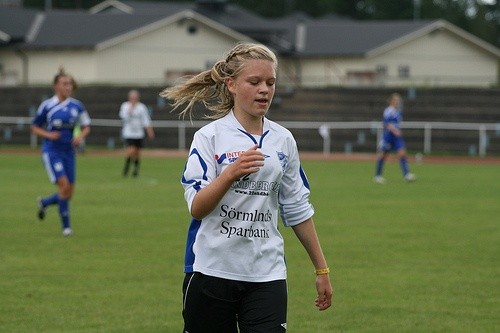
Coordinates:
158 43 333 333
373 92 416 184
119 88 154 177
30 72 92 237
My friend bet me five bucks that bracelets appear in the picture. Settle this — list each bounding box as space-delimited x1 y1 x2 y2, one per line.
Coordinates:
314 267 330 275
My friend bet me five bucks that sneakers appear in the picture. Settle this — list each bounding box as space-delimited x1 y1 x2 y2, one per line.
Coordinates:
373 172 385 185
60 226 73 236
121 170 139 178
404 174 415 183
39 198 46 220
